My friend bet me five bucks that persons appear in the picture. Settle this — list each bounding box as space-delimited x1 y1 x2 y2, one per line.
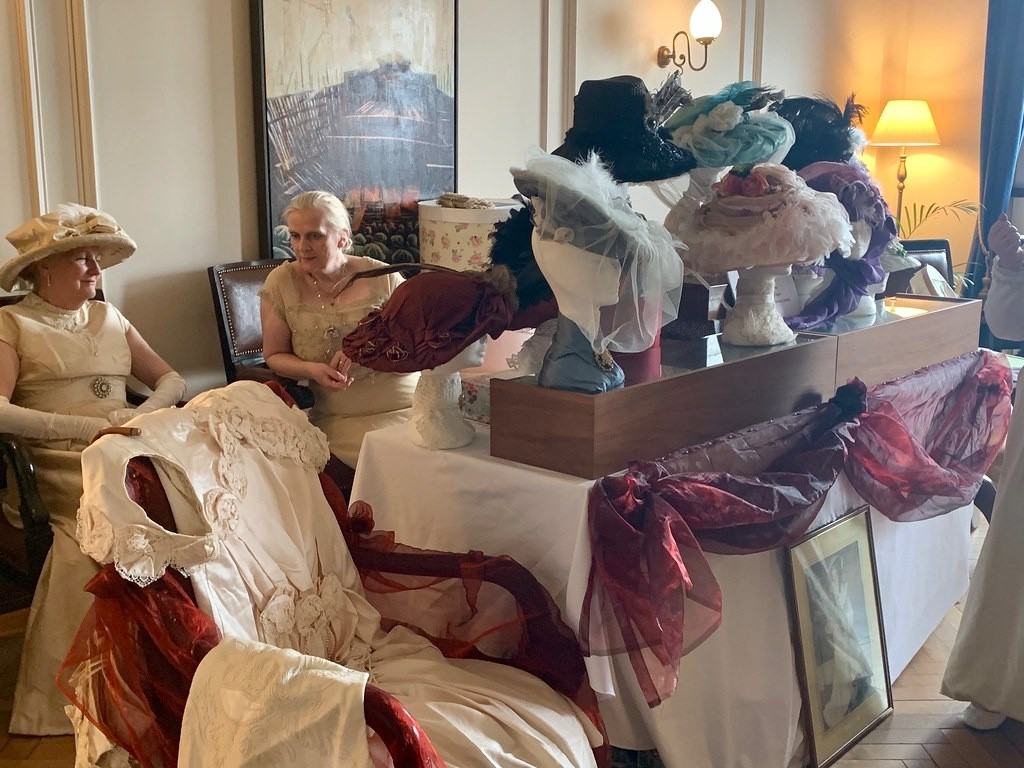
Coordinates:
531 223 625 396
660 99 890 347
938 212 1023 730
395 268 487 452
261 190 424 480
0 210 187 550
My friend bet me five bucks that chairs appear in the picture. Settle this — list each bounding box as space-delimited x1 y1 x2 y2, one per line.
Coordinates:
1 294 187 582
207 259 354 494
78 384 610 768
889 238 954 302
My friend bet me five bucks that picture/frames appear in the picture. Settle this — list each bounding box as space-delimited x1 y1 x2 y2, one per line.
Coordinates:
250 0 458 257
783 504 894 768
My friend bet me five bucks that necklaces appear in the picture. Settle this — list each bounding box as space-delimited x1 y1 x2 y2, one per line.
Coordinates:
313 259 344 362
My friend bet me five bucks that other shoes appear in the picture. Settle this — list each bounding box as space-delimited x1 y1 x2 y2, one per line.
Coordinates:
963 704 1006 730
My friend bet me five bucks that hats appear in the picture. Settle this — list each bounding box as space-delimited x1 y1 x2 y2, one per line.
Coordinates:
674 161 856 276
330 262 518 373
509 145 691 356
0 202 137 293
796 161 887 229
550 75 870 186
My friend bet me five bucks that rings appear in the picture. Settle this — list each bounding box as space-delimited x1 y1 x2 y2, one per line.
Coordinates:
339 361 345 365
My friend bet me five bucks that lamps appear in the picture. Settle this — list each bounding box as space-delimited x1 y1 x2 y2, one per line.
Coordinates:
869 97 940 236
657 0 722 74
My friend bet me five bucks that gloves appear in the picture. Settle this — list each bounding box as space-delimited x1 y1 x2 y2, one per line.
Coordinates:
0 396 112 444
109 372 188 427
